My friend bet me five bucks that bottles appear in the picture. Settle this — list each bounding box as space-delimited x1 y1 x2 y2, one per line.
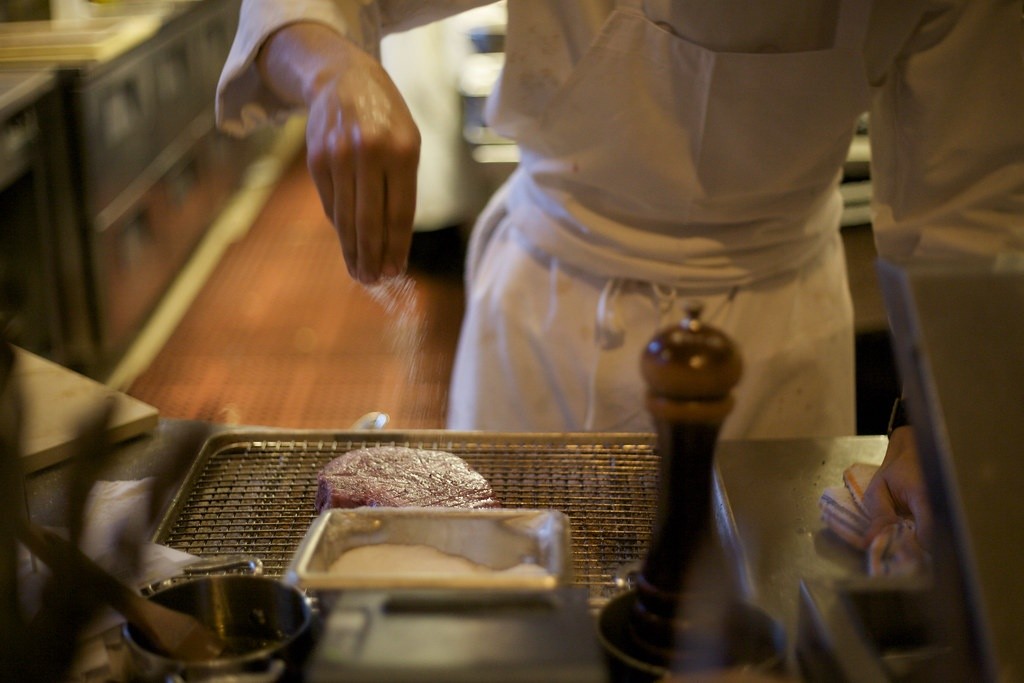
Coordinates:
631 304 750 667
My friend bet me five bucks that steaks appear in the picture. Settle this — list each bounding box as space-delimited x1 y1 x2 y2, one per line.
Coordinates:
314 445 501 510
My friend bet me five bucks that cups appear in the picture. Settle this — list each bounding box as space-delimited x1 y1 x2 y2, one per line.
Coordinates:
122 555 311 683
597 589 791 683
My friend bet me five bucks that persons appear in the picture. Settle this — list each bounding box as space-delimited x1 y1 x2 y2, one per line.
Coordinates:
216 0 1023 439
